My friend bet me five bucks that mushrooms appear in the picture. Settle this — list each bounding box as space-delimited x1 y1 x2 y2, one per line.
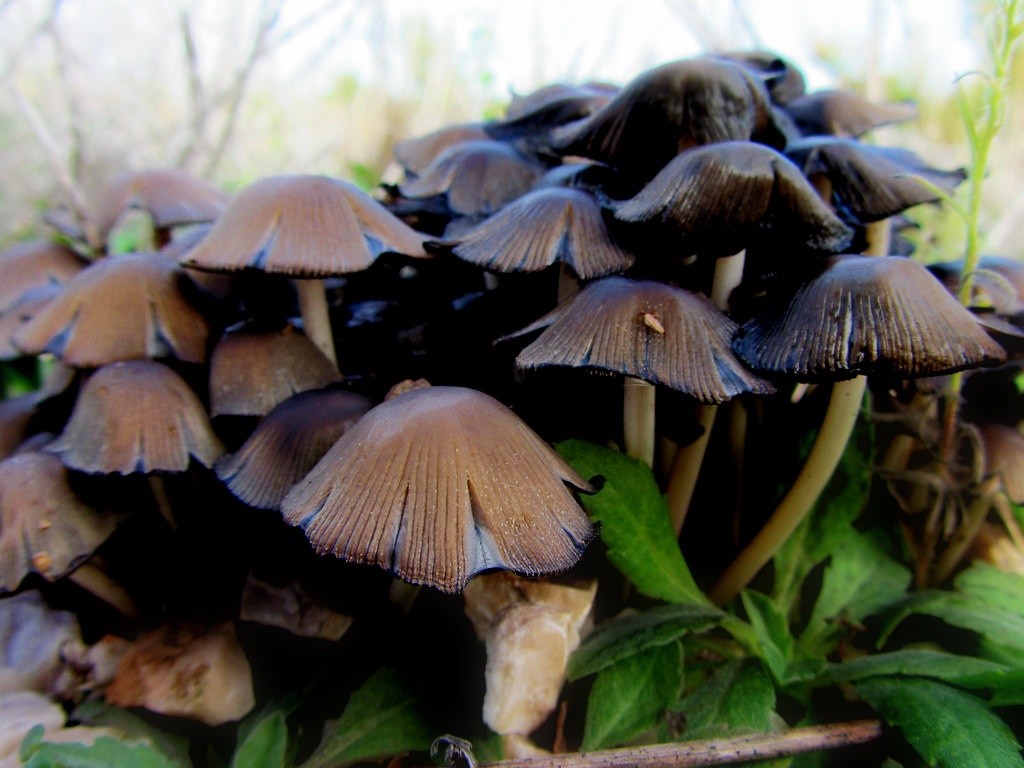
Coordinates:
0 53 1024 752
